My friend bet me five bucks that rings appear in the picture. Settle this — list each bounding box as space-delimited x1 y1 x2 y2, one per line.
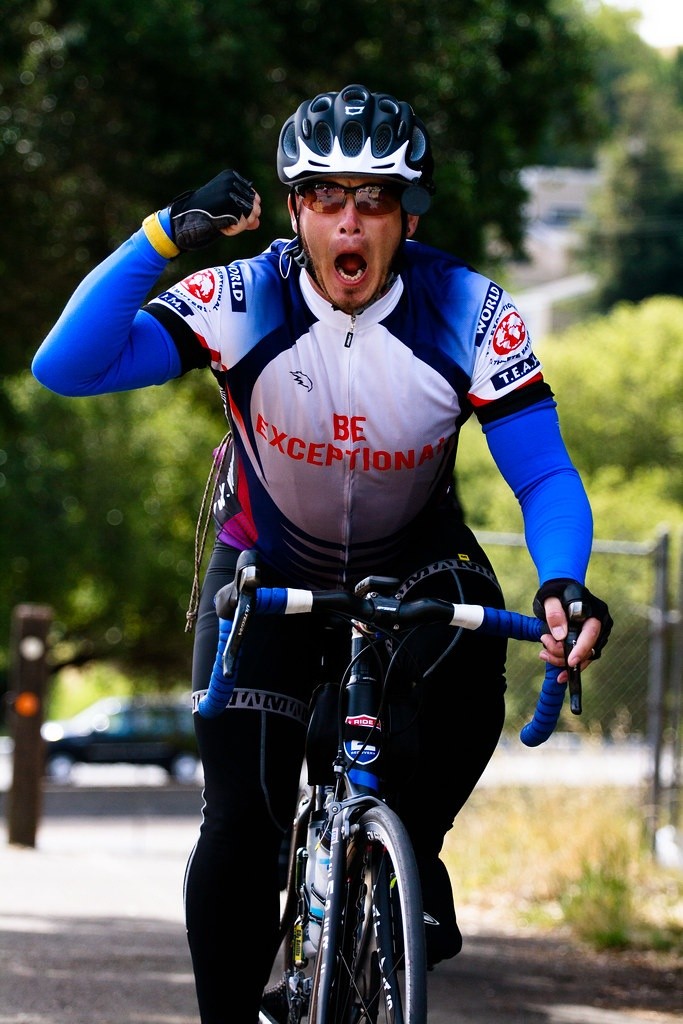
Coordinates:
590 648 595 654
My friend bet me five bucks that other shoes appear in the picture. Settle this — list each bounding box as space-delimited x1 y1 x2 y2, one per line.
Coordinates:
258 979 309 1024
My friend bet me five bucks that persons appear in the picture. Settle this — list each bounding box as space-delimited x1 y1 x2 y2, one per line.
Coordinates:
31 82 613 1024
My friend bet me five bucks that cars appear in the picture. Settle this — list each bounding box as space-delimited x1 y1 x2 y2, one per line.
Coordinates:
42 698 203 785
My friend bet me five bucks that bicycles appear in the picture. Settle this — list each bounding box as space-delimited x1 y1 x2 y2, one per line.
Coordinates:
195 549 583 1024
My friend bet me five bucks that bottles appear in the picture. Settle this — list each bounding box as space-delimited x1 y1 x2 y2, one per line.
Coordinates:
308 821 333 951
305 808 327 905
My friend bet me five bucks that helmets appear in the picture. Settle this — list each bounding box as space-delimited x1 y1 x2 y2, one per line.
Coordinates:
276 83 436 215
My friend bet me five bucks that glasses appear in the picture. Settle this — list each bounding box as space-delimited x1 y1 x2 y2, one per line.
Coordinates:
293 177 407 216
